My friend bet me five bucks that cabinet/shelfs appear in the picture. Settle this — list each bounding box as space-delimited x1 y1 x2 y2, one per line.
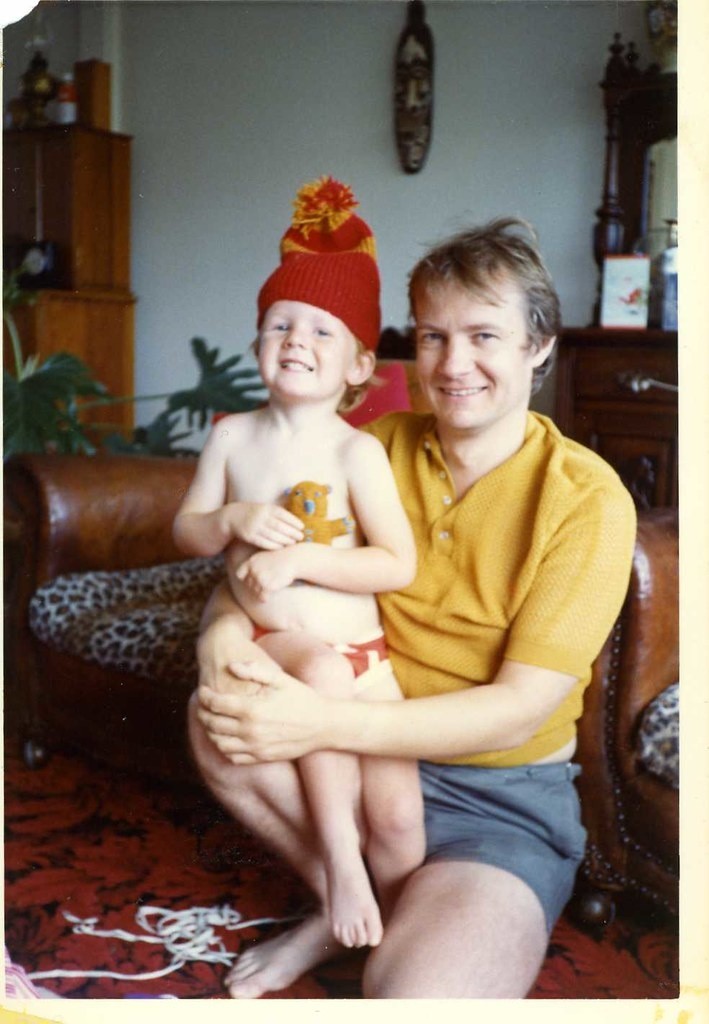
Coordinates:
552 328 679 509
3 120 139 451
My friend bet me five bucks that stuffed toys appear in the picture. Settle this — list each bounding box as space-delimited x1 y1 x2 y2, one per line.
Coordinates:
281 479 358 547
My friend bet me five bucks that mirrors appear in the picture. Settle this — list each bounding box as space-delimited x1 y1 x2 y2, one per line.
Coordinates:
639 127 677 327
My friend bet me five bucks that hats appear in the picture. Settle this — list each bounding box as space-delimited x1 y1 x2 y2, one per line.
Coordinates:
257 174 382 350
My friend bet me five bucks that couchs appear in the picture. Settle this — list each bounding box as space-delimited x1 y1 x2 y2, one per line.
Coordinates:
574 507 680 941
3 454 242 790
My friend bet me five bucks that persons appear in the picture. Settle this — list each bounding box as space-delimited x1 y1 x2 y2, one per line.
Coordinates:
170 174 430 949
184 217 640 999
